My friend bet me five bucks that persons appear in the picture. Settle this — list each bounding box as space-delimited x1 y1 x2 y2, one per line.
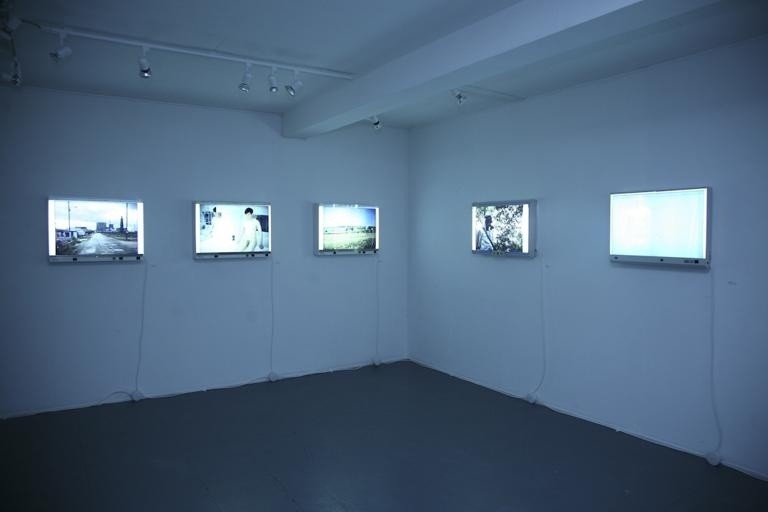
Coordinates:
476 215 493 251
120 228 125 240
125 228 129 240
206 207 221 239
238 208 263 251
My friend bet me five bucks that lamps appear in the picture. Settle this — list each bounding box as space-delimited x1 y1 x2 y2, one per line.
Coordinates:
2 5 24 87
34 24 356 104
448 86 468 105
366 115 385 132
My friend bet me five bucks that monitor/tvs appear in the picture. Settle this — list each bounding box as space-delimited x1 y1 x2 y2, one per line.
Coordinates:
47 194 144 264
472 199 537 258
314 202 382 256
609 186 711 272
192 200 271 260
